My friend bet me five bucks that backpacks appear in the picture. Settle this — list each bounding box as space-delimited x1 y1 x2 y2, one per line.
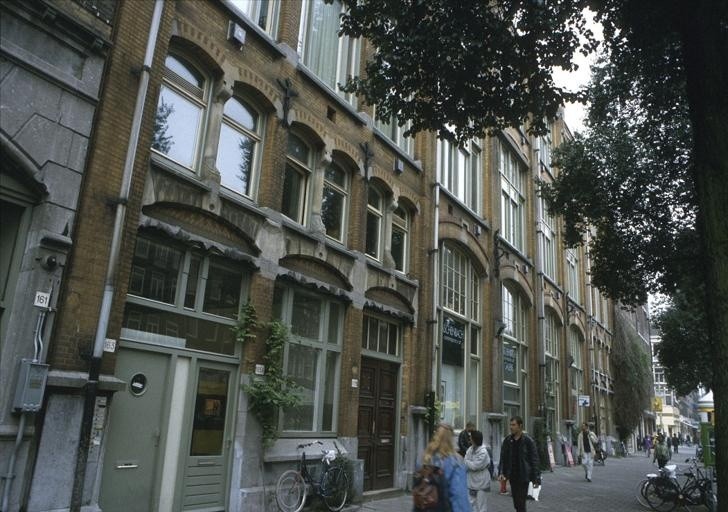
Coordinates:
412 462 452 512
487 459 495 481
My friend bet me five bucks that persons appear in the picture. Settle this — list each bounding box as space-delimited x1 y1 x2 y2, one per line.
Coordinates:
636 430 692 457
412 422 474 511
462 429 492 511
497 415 542 511
485 444 495 480
576 421 600 482
652 434 672 469
458 420 475 455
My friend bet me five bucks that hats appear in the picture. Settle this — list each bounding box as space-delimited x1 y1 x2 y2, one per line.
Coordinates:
470 429 483 446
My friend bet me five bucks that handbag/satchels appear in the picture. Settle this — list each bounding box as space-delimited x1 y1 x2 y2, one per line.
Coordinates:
662 454 670 464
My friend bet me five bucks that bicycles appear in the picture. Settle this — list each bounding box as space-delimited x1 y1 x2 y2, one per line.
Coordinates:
592 439 608 464
631 452 719 512
272 438 351 512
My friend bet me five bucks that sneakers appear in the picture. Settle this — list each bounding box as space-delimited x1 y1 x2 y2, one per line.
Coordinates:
585 472 592 482
